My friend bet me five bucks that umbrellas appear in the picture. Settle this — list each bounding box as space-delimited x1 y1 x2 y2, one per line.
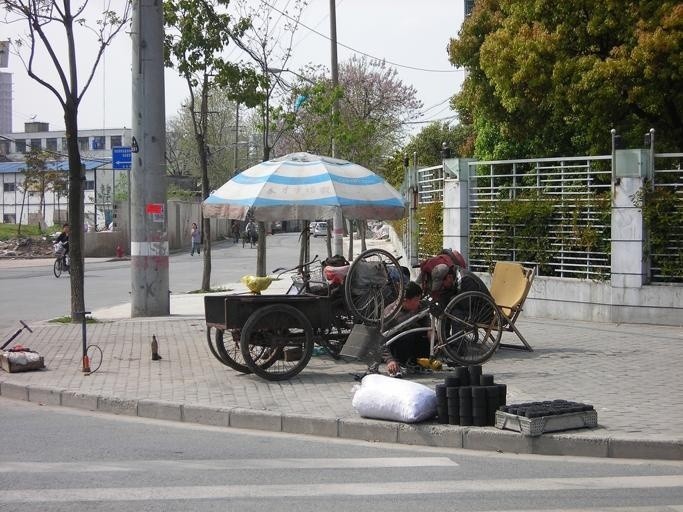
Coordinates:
201 152 408 287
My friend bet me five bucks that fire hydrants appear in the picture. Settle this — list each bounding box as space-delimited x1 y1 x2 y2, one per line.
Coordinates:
116 244 123 257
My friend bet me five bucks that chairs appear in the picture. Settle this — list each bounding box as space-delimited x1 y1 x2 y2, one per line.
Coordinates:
472 260 536 352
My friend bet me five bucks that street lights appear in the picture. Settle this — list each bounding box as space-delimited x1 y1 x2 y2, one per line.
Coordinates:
262 0 344 259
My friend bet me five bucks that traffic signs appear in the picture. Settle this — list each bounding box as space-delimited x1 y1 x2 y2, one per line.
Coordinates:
111 145 132 171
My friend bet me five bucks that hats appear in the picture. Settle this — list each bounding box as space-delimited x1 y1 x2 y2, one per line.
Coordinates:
430 264 449 292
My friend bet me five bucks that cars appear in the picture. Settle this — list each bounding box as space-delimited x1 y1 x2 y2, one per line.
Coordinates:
309 222 327 238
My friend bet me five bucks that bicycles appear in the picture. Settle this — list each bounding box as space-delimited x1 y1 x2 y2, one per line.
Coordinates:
53 244 70 278
339 249 503 382
243 255 326 295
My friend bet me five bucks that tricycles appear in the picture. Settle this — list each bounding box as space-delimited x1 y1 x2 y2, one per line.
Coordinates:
242 230 258 247
204 247 404 382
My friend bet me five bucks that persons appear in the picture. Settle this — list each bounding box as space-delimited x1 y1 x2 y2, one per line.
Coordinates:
431 263 495 367
53 224 70 271
190 223 201 256
380 281 437 375
231 219 240 244
246 218 259 246
271 221 276 235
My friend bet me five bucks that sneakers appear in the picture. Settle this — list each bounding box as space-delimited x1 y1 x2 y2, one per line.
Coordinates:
389 359 434 377
